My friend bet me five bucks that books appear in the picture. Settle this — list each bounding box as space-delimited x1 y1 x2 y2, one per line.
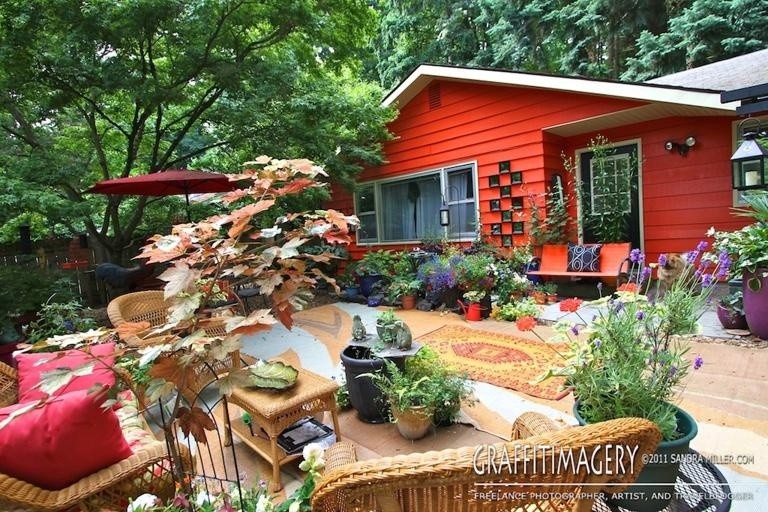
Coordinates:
259 414 334 457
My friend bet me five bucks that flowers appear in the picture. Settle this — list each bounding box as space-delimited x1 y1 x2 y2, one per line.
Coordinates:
127 442 326 512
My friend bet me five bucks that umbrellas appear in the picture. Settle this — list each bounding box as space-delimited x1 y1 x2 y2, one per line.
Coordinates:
78 167 238 222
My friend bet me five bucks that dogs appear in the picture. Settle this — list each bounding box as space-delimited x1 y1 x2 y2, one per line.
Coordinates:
657 253 697 300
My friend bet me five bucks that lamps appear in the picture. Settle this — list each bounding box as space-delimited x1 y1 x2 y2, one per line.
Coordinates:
439 186 460 253
730 113 768 191
665 136 696 154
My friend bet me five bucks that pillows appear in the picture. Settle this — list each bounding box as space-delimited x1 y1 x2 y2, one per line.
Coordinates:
0 384 134 490
15 342 123 412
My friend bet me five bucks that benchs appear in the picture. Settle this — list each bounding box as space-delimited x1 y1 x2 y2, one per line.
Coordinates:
0 361 196 512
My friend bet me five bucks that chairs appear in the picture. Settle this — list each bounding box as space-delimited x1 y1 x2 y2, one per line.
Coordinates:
107 290 240 408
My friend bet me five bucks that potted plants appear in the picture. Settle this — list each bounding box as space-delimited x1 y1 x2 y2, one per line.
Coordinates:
716 291 749 329
334 236 733 512
704 221 768 341
